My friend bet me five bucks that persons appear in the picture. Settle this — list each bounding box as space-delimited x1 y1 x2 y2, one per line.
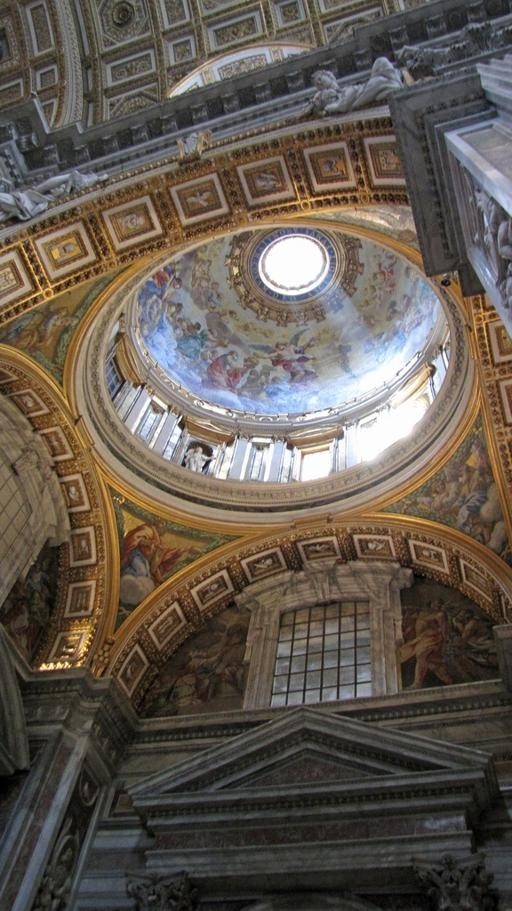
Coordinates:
468 189 511 309
306 53 406 112
393 21 512 70
0 169 110 223
